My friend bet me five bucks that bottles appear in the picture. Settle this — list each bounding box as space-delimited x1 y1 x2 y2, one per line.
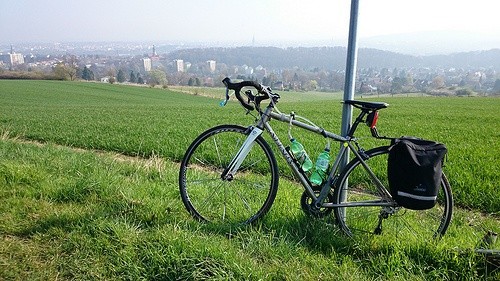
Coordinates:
310 147 330 186
290 138 313 171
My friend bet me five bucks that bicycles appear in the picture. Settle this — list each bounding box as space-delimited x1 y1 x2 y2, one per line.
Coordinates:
179 78 454 248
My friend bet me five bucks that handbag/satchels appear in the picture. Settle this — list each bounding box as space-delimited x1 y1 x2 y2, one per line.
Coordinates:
388 136 447 210
